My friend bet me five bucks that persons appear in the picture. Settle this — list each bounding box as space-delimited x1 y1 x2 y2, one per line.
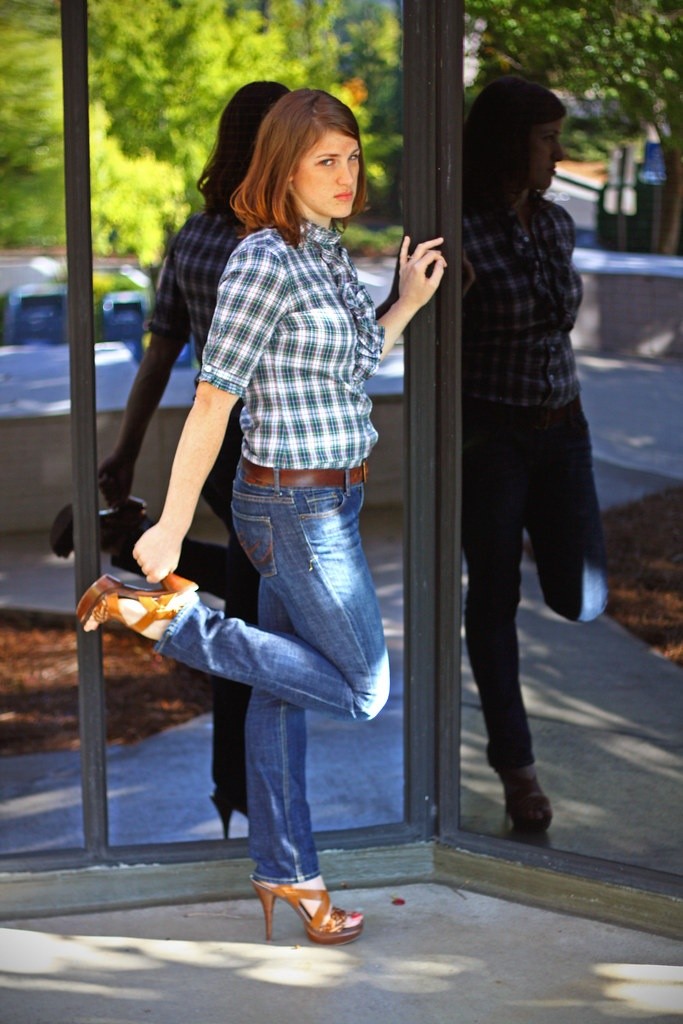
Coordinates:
377 78 609 835
78 88 447 945
48 79 291 839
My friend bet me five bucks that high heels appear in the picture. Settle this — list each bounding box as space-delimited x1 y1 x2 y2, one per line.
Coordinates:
77 572 199 634
249 874 364 947
521 527 536 562
50 495 146 558
499 762 554 835
209 784 248 839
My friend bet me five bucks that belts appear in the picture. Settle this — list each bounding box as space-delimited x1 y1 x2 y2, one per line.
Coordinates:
239 454 368 486
462 394 582 430
